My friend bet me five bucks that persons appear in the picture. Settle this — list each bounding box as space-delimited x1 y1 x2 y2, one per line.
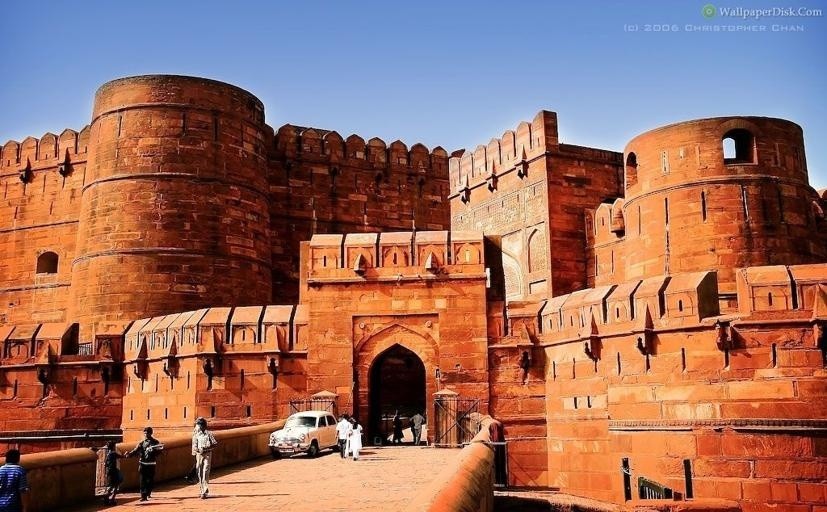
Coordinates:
390 409 403 445
190 416 217 498
409 411 425 444
0 447 30 512
347 416 363 461
123 427 165 502
102 440 125 506
335 412 350 460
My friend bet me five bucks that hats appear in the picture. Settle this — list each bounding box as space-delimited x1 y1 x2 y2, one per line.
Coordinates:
195 417 206 424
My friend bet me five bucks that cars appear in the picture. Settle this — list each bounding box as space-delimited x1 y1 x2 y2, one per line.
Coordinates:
266 408 342 461
